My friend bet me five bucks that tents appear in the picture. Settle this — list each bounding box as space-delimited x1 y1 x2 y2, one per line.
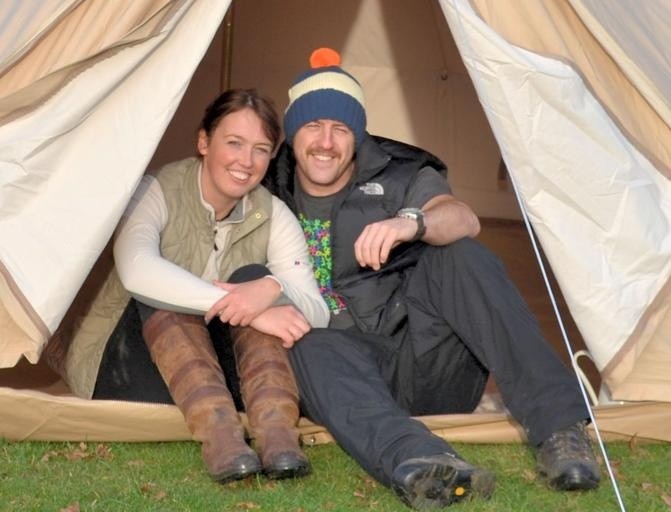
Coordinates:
2 0 670 445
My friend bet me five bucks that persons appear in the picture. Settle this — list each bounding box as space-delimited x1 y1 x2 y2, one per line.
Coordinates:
38 88 332 481
257 47 603 511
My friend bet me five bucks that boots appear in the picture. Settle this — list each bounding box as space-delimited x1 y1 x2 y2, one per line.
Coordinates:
229 317 314 478
140 307 265 483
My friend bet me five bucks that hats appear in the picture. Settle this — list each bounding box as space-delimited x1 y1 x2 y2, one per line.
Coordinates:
283 65 368 160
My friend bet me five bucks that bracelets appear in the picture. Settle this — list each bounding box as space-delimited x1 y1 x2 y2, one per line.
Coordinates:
393 205 427 245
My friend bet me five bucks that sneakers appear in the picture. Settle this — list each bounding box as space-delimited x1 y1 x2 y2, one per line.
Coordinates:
533 418 603 490
391 452 498 511
